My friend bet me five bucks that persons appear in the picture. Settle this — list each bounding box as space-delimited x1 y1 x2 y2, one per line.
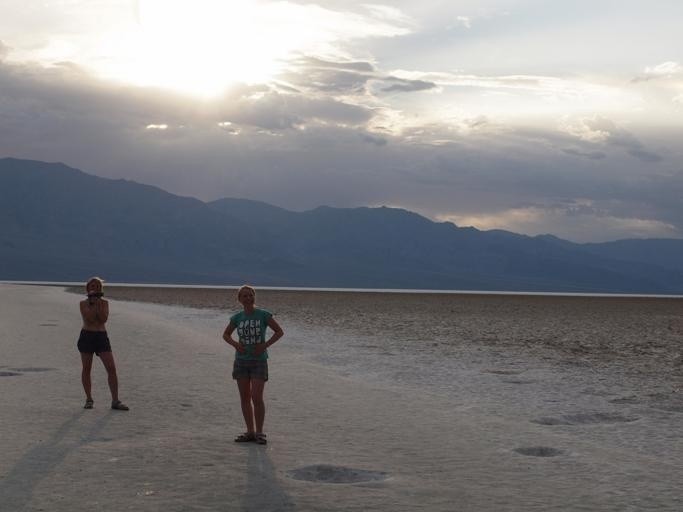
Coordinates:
222 286 284 444
76 277 131 410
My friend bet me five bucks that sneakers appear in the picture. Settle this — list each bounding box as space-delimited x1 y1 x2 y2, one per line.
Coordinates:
110 400 130 410
83 399 93 409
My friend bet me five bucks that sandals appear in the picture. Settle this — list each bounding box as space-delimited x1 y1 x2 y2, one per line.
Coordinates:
233 432 255 442
254 431 267 445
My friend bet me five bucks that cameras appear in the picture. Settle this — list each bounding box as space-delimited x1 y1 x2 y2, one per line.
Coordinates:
93 291 104 298
